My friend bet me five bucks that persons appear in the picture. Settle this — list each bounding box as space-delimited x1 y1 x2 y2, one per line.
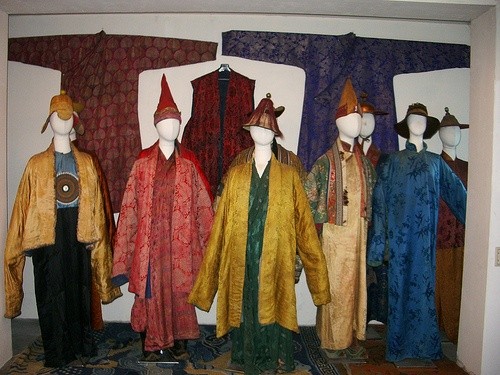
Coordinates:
366 102 468 363
3 94 123 365
109 74 216 359
298 77 378 350
434 107 470 345
357 101 391 323
186 96 331 375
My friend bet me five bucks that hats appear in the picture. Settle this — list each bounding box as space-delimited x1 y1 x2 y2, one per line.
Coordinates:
241 94 283 139
247 105 286 118
39 90 86 135
436 107 469 130
154 72 183 126
356 91 389 117
393 102 441 140
335 75 364 119
